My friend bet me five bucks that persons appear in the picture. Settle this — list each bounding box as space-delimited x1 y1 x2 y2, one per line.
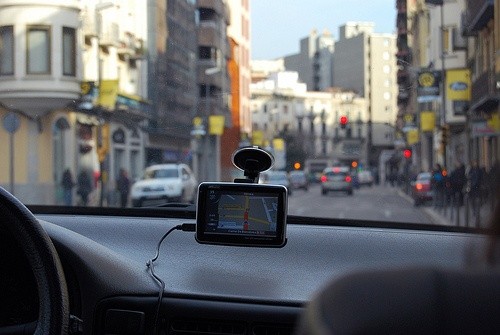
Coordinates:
77 171 92 206
62 169 75 207
429 159 500 229
102 169 129 209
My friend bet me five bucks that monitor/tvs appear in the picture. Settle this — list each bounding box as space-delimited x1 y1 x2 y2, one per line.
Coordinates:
195 182 287 246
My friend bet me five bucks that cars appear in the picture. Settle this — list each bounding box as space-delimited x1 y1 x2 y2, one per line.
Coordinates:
257 160 377 196
411 170 434 207
320 165 354 195
130 161 200 207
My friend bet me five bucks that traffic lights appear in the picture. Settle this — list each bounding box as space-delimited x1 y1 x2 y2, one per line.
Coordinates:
340 116 346 129
